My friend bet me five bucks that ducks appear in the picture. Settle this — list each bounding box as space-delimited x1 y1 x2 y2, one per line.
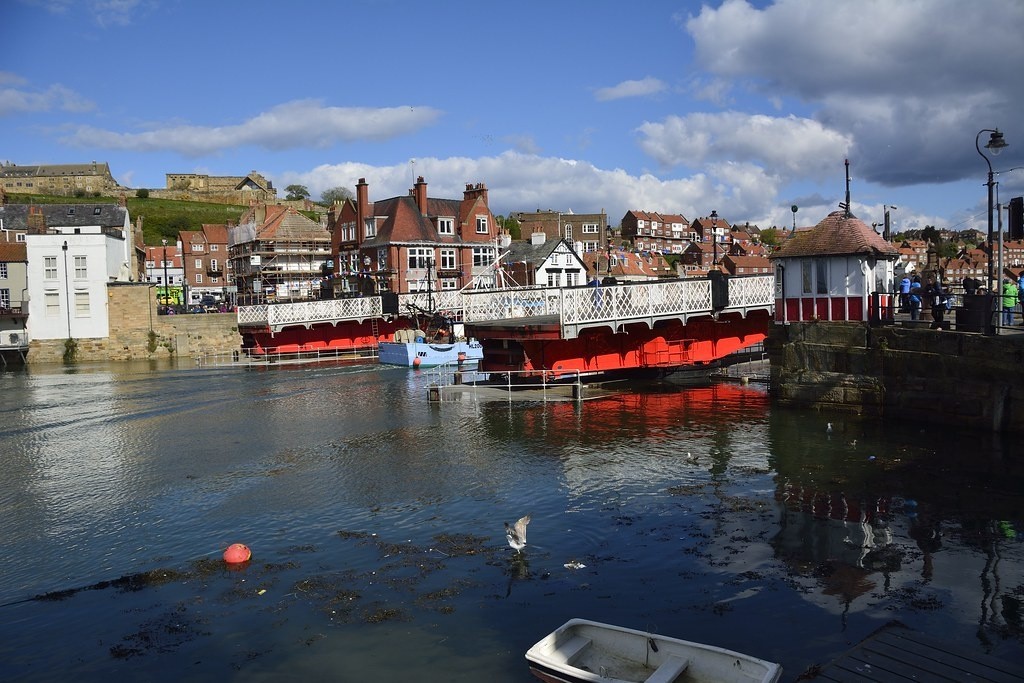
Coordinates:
826 423 832 432
685 452 699 465
846 439 859 446
504 513 531 554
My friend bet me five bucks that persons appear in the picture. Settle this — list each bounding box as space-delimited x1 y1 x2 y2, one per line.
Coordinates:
1002 279 1018 326
922 275 944 331
191 304 227 314
898 276 911 313
1018 270 1024 326
940 277 953 314
159 305 175 315
893 272 899 297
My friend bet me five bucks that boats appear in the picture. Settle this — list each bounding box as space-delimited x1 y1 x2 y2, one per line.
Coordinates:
379 328 484 367
525 617 783 683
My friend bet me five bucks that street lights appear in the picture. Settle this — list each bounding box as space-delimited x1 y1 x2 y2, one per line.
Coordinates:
881 204 898 245
603 224 617 285
975 128 1010 291
708 210 723 271
